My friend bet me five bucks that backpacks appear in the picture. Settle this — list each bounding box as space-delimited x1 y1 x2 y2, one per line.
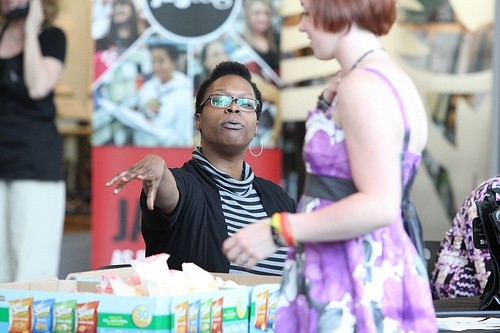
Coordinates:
426 177 500 306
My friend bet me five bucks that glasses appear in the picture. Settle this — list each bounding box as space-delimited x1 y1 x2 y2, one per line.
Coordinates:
199 93 262 113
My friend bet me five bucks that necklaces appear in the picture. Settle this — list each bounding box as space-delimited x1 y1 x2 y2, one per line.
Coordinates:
335 48 374 85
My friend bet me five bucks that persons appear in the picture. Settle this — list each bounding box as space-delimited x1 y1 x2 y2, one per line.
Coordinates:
92 1 147 81
104 61 298 290
0 0 67 282
427 174 500 303
192 37 284 155
237 0 280 82
112 42 197 151
222 0 440 333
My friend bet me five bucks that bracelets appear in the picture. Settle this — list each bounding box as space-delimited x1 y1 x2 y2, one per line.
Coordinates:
269 211 300 249
